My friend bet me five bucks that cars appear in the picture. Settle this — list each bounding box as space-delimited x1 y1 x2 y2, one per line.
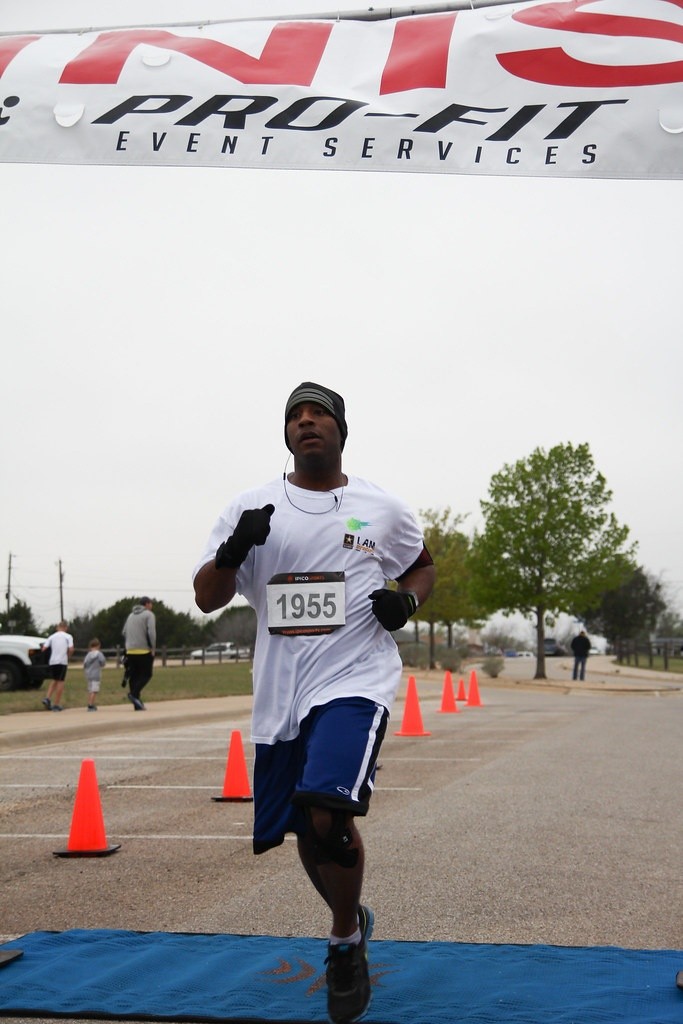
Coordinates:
190 642 251 661
516 651 533 657
589 646 601 655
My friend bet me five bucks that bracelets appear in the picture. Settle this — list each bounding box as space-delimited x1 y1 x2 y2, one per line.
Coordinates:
406 592 418 607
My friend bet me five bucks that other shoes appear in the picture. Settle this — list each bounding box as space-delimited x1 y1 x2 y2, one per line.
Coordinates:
51 705 63 712
122 680 126 688
42 697 52 711
88 705 97 711
129 692 145 710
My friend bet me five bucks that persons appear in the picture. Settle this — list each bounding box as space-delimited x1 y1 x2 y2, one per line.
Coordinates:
83 639 105 711
121 596 156 711
190 382 436 1024
571 632 590 681
41 621 74 711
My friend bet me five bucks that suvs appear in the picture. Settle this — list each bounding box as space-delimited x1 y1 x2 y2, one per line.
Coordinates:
0 623 53 693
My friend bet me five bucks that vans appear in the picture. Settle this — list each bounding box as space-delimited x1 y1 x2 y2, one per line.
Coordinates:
544 638 566 657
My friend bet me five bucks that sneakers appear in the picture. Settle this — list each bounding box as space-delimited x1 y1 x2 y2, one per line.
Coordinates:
358 903 375 960
324 926 373 1024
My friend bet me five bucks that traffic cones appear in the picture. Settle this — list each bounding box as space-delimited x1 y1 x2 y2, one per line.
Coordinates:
463 670 485 708
211 730 254 802
435 671 462 713
51 756 124 857
454 679 468 701
393 675 432 737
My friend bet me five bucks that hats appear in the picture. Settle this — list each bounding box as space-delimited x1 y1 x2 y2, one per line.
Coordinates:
284 382 348 454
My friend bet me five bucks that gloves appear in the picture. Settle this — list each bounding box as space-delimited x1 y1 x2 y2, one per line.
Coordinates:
215 504 275 569
368 589 417 631
